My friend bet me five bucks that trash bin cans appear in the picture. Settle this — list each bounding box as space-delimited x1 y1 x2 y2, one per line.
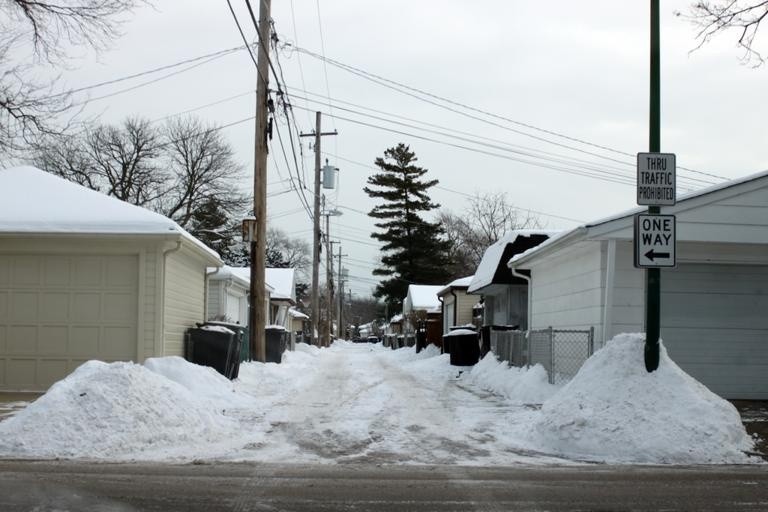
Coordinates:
265 328 291 363
442 326 480 366
185 321 248 380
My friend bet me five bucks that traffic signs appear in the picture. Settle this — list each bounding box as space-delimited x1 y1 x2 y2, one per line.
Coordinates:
638 154 675 204
639 215 675 266
